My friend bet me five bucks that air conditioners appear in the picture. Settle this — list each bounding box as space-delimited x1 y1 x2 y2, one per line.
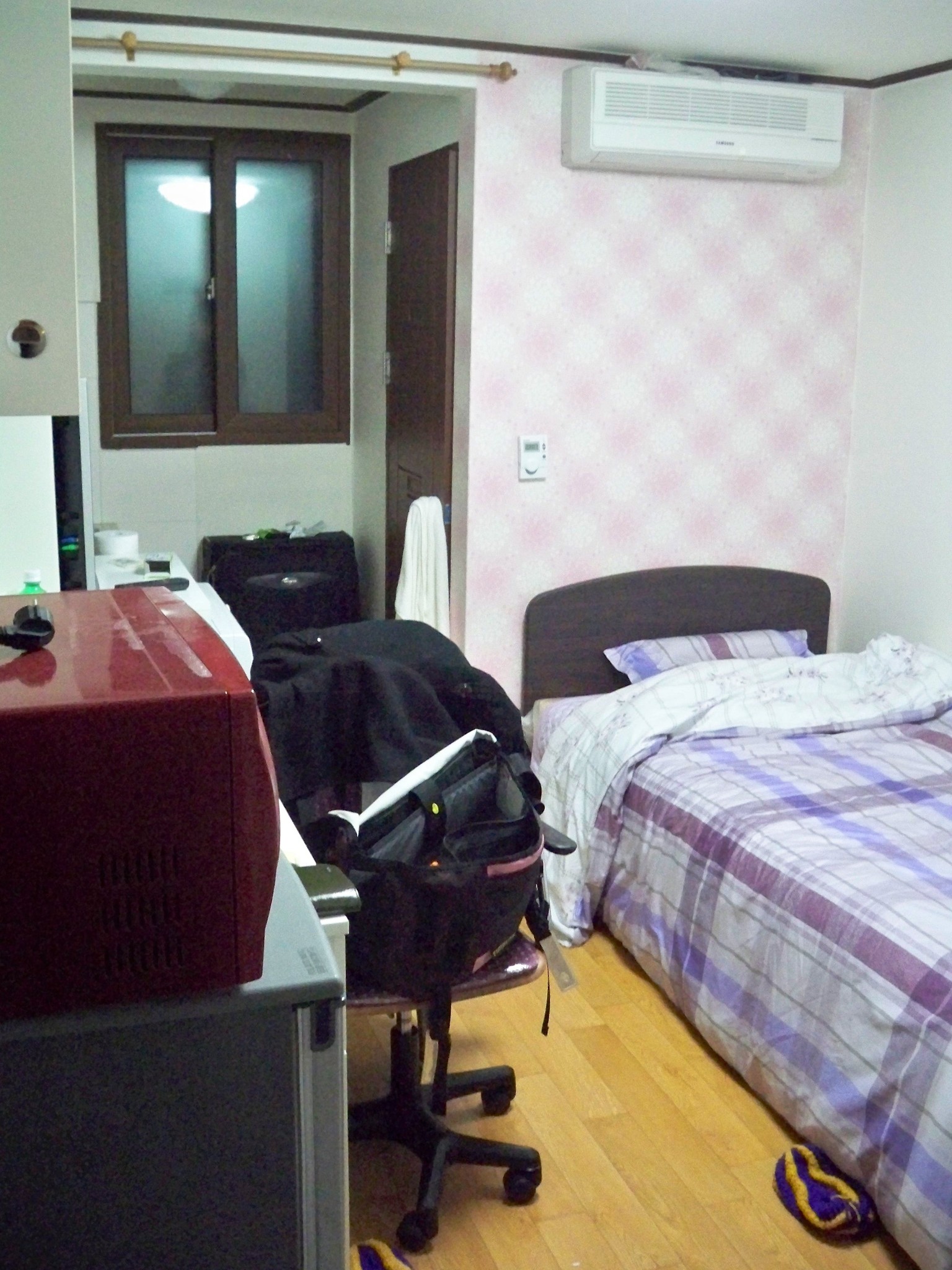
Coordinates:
562 65 843 182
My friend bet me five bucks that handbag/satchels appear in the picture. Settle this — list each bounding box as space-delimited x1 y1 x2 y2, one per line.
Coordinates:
345 740 545 999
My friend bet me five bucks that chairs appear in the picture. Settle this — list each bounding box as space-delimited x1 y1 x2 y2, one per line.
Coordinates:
251 620 578 1252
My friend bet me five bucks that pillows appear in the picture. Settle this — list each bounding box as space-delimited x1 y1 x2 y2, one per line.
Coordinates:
603 628 816 684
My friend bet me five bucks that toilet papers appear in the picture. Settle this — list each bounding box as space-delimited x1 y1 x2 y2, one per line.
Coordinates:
93 529 140 559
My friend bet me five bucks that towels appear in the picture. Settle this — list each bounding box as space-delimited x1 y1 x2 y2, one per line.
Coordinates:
390 492 451 639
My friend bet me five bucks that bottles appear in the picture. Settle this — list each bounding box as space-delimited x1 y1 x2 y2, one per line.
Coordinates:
19 569 46 595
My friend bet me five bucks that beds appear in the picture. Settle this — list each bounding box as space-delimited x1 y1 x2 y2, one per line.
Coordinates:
522 566 952 1270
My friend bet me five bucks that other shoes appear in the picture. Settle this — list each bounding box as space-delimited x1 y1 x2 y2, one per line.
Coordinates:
775 1143 874 1240
350 1239 413 1270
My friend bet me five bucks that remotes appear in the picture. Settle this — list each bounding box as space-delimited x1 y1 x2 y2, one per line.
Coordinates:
115 579 191 590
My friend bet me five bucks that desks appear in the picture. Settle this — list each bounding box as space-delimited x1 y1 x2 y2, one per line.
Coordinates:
93 549 350 1270
0 848 344 1270
203 530 363 629
